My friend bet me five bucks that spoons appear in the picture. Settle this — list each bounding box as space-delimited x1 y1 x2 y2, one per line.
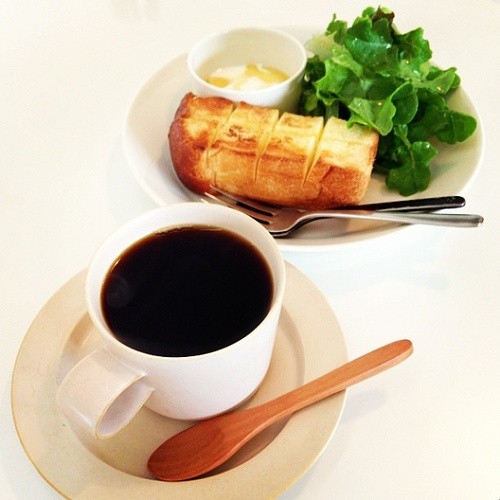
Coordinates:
147 338 413 482
199 184 485 229
237 196 465 236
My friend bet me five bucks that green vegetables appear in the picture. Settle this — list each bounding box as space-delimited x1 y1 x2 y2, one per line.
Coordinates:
301 6 478 198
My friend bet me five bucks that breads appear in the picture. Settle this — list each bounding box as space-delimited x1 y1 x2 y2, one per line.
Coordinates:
169 93 379 212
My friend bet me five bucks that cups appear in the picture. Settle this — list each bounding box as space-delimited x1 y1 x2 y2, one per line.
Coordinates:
59 201 285 438
189 26 308 116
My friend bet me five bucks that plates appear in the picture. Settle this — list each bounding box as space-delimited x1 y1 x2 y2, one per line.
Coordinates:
11 263 349 500
127 23 483 246
